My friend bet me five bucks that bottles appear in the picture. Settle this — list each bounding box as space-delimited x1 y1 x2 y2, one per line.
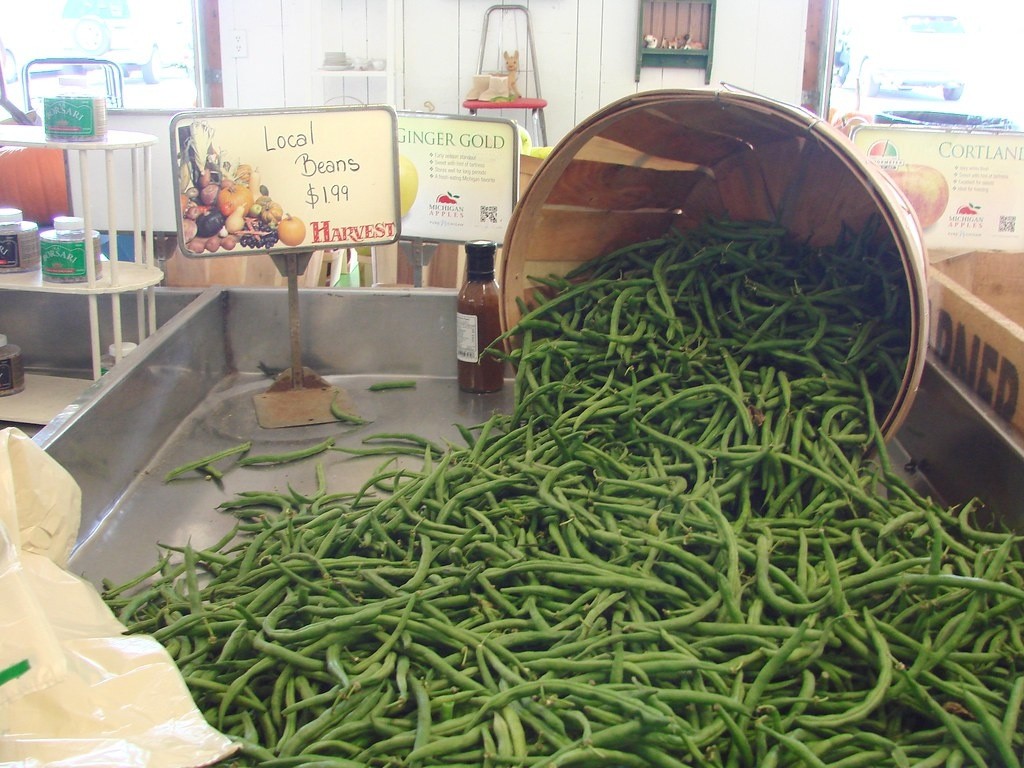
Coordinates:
100 342 138 375
0 333 26 397
42 75 108 143
454 240 506 394
0 207 39 274
40 217 103 283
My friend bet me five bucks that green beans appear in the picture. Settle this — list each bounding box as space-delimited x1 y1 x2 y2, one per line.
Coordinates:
89 208 1024 768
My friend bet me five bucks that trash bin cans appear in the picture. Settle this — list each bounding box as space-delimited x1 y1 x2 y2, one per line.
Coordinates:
875 109 1014 130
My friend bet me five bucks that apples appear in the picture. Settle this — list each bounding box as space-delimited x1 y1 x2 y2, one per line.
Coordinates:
883 161 949 226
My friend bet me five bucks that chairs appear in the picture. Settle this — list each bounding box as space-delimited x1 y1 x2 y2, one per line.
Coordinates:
462 4 549 148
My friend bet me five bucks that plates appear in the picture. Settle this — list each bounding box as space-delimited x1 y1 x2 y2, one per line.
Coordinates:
317 51 354 71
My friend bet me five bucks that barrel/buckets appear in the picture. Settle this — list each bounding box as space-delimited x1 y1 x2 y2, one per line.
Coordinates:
500 85 930 468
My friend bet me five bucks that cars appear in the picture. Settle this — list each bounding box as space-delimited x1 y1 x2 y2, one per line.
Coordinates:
857 9 1016 134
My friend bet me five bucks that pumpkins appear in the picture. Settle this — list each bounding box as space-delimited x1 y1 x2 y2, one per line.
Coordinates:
0 145 75 227
181 164 306 247
827 106 873 137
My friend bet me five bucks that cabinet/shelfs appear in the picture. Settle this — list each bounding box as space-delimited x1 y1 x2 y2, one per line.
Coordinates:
0 122 159 434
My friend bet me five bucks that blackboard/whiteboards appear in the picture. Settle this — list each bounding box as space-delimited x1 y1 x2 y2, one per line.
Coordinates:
169 105 403 258
394 110 520 248
